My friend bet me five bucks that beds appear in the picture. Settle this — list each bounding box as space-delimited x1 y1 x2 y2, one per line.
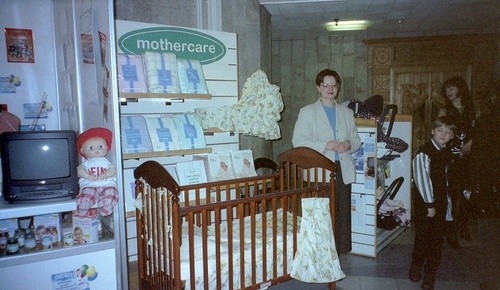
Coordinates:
134 146 338 290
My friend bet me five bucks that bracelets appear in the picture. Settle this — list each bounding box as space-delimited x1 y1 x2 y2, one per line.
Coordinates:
348 141 352 151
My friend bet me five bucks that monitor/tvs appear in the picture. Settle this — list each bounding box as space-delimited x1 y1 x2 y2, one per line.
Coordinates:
0 130 80 203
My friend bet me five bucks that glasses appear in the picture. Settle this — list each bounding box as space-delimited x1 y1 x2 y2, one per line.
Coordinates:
320 83 337 88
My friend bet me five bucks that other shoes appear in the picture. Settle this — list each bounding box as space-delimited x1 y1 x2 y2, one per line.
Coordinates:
421 270 436 290
409 264 422 282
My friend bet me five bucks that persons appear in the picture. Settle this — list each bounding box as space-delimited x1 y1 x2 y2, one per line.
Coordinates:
35 225 47 241
76 127 118 217
291 68 361 257
74 227 87 244
411 78 476 290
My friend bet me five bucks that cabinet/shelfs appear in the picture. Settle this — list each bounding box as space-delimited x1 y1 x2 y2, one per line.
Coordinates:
0 195 121 290
118 92 215 219
